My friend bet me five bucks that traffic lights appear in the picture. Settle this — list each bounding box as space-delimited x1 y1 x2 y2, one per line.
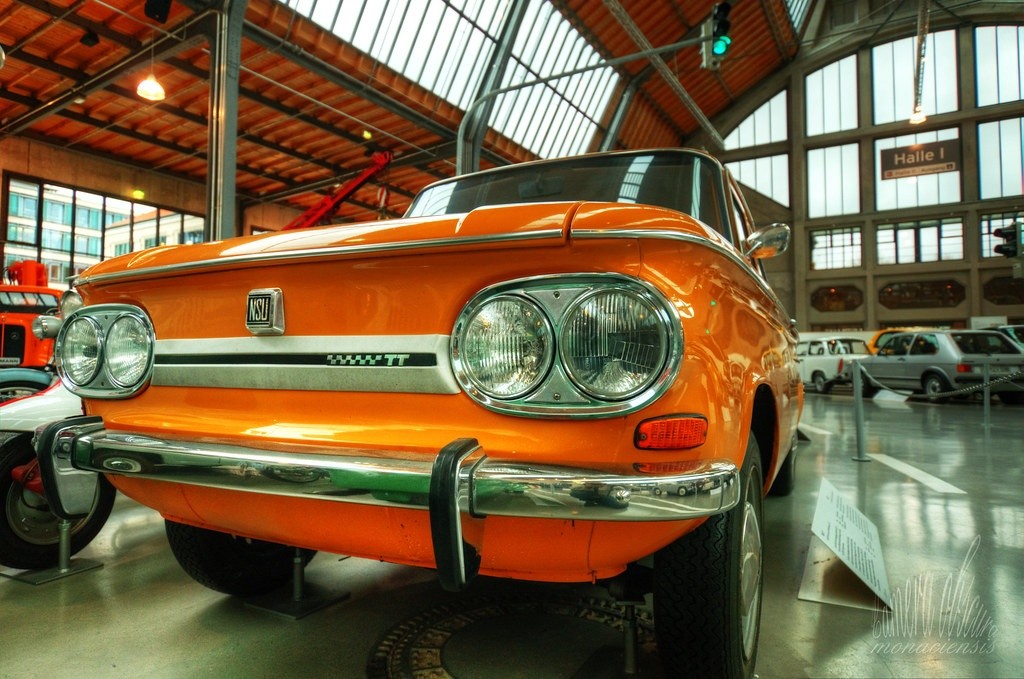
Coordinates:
713 1 731 56
993 222 1021 258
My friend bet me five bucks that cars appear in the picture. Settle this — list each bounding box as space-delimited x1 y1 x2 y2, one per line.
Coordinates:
865 327 941 356
54 141 803 679
795 337 874 393
848 330 1024 404
0 259 118 569
958 325 1024 354
